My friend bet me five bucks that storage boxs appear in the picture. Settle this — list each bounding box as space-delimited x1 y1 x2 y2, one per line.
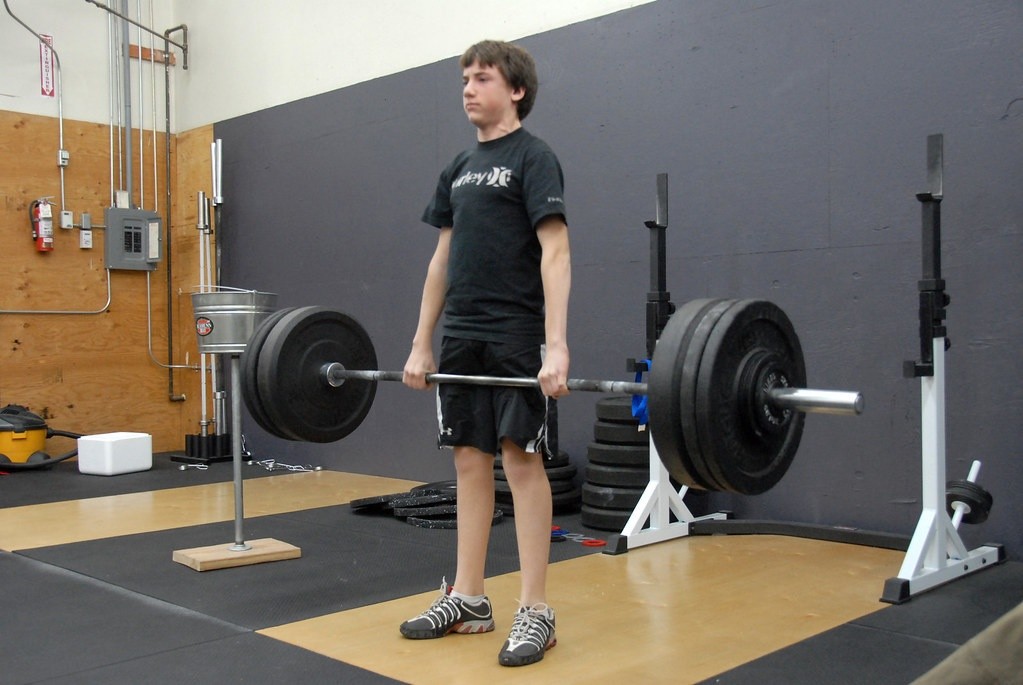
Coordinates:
77 432 152 475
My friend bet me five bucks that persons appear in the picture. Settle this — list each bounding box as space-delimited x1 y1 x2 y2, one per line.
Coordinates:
400 41 571 667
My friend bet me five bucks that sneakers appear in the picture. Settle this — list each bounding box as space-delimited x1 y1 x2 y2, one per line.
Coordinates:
400 575 495 639
498 597 557 667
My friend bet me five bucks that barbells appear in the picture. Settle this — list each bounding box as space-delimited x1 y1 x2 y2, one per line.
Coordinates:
237 295 866 495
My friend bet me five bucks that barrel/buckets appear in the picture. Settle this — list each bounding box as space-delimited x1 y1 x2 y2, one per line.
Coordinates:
191 286 278 355
